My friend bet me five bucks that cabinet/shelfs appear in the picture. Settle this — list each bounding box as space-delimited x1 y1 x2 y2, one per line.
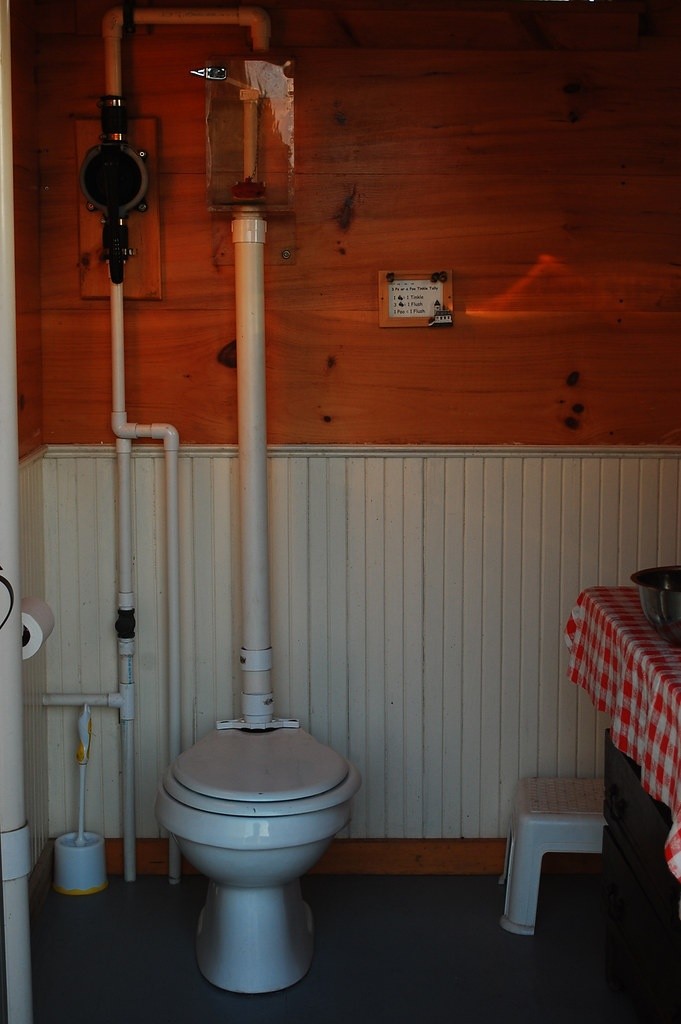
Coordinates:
563 587 681 1024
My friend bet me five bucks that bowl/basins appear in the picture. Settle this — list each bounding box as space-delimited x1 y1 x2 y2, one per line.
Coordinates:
630 565 681 645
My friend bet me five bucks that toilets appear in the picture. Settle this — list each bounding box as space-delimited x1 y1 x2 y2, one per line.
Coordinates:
157 728 360 994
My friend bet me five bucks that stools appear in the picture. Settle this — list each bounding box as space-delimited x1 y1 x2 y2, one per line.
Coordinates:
497 778 609 936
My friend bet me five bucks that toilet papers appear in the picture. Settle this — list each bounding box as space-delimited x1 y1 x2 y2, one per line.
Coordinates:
20 596 55 661
